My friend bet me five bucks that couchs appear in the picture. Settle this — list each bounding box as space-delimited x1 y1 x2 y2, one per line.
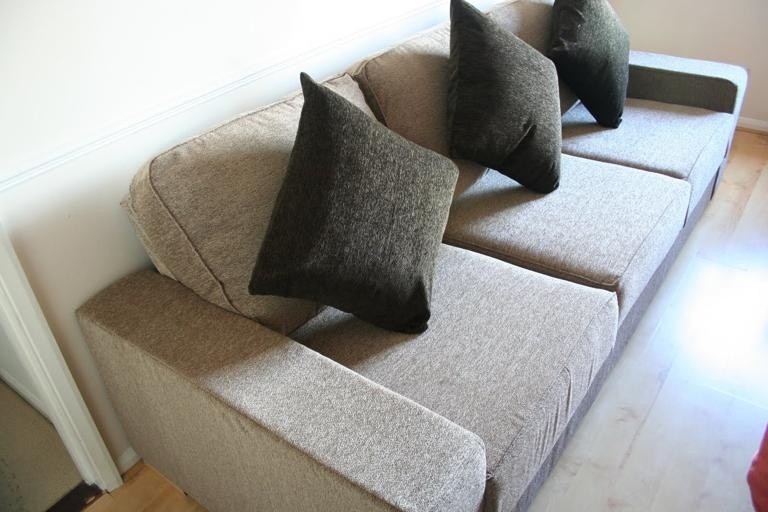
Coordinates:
74 49 748 512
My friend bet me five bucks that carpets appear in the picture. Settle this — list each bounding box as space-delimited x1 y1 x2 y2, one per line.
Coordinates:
0 377 103 512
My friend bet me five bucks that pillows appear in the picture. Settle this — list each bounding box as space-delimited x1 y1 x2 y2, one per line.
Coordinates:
446 0 563 194
548 0 631 129
248 72 460 334
